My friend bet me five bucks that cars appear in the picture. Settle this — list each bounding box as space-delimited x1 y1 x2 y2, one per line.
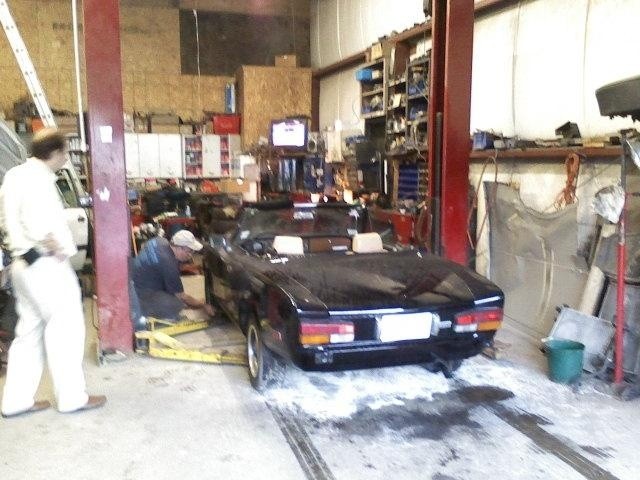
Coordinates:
0 164 89 312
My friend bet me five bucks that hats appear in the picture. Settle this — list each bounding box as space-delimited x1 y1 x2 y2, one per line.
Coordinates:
171 230 204 251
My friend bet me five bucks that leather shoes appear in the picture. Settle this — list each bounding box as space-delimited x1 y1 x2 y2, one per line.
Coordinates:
2 400 50 417
78 396 106 410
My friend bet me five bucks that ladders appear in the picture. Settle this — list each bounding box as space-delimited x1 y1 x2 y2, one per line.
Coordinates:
0 0 90 207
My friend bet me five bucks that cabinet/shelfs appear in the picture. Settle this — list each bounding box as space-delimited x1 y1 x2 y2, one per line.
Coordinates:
56 132 88 181
358 56 432 158
125 133 242 179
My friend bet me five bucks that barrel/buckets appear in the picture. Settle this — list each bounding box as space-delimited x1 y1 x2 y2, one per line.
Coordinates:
544 338 585 384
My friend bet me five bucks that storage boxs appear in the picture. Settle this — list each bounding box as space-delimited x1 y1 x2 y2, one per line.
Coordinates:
125 113 240 134
274 53 302 69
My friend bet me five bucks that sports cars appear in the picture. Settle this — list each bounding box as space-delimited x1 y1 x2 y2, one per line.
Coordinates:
199 200 506 394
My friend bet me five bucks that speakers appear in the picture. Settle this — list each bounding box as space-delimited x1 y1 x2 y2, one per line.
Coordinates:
308 132 318 152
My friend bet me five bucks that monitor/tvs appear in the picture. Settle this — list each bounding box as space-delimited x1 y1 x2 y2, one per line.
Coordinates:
270 119 308 150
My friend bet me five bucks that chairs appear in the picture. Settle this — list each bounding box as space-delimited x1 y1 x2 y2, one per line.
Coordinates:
345 232 389 255
262 235 305 259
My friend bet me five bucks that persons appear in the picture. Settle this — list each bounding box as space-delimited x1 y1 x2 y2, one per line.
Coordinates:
141 178 430 242
130 228 220 328
1 123 107 422
